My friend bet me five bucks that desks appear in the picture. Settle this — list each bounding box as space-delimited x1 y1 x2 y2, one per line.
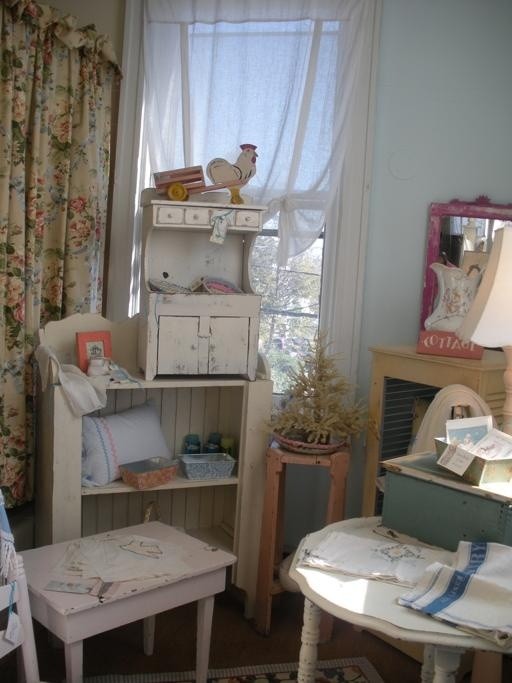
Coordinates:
285 514 512 683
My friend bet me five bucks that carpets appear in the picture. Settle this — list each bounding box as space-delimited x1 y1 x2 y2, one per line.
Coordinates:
63 655 384 683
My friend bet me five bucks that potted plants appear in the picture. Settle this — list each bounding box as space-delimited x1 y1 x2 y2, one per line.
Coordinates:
265 325 381 455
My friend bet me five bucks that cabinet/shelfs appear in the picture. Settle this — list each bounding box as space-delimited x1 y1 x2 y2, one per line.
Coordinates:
33 310 276 621
139 202 266 381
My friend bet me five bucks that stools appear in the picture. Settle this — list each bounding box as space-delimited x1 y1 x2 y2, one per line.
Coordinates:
17 519 238 683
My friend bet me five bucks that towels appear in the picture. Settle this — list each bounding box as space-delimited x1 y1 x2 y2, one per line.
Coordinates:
35 343 110 416
397 539 512 648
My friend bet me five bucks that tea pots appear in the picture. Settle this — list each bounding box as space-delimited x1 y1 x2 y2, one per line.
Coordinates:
419 264 487 333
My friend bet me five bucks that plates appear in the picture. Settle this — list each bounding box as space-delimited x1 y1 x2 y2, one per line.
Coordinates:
272 427 350 455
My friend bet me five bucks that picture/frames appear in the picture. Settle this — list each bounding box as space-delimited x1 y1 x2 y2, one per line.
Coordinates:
75 330 113 375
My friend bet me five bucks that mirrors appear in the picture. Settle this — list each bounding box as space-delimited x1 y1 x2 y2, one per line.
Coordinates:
419 192 512 331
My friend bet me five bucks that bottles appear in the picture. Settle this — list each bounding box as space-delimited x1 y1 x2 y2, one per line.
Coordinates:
183 433 237 455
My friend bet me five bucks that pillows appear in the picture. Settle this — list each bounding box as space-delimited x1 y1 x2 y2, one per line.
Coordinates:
80 395 176 487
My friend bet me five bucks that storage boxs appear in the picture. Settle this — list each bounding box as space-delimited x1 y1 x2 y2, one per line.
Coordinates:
381 452 511 553
